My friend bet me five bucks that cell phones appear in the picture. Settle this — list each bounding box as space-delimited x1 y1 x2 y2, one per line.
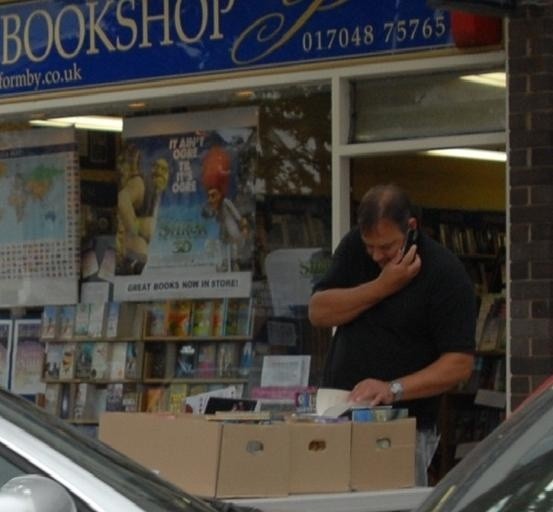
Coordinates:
403 227 418 257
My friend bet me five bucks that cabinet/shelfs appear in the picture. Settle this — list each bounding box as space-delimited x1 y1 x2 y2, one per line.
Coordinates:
418 206 505 487
34 291 274 426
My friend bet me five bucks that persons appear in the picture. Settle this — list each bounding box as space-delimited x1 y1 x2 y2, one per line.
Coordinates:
200 187 252 271
306 183 478 482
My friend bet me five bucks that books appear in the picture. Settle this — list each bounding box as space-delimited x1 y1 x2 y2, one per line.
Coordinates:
414 213 506 395
45 213 326 424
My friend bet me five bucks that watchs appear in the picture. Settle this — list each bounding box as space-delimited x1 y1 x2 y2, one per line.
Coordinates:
388 379 405 401
